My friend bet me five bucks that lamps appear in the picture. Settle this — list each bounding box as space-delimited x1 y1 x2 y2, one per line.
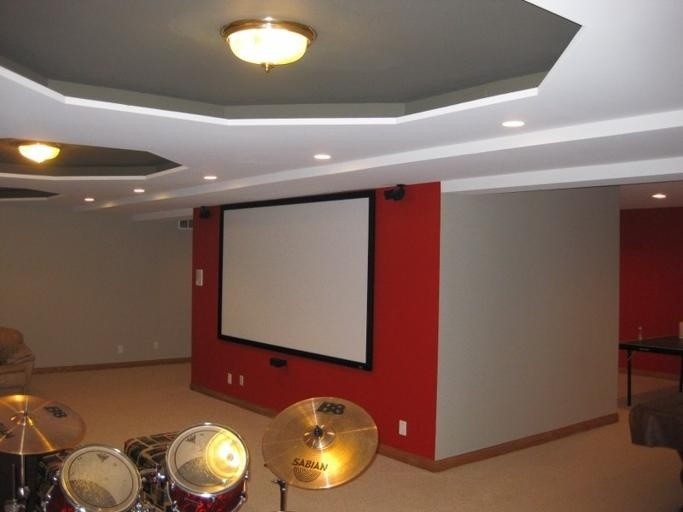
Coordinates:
219 18 313 73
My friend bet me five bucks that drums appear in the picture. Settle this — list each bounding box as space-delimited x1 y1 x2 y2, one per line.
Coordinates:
161 420 249 512
43 442 142 512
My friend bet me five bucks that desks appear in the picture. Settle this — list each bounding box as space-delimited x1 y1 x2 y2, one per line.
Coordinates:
615 336 682 407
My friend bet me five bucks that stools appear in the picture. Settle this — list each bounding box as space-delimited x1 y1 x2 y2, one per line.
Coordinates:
124 429 182 512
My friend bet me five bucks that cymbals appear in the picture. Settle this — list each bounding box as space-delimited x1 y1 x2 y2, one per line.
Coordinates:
0 394 87 456
262 396 379 491
